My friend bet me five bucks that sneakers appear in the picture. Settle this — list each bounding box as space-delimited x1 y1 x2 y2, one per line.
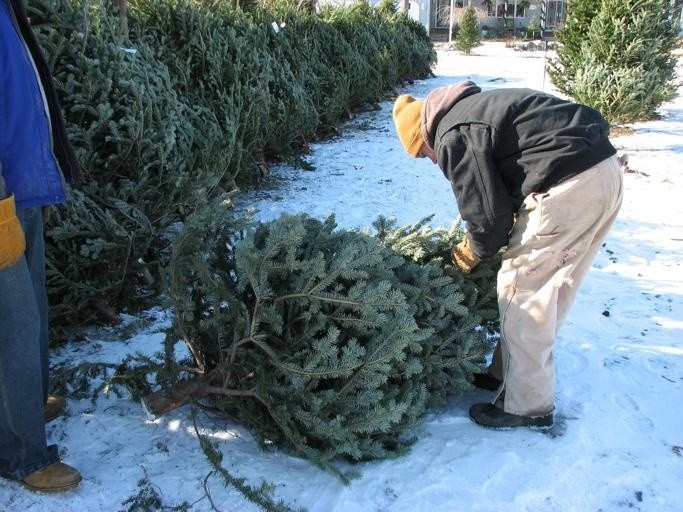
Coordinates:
43 394 66 422
469 403 554 429
470 370 503 392
15 460 82 492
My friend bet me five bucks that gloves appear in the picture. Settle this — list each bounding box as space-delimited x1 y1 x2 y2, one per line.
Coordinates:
0 192 25 269
451 235 482 276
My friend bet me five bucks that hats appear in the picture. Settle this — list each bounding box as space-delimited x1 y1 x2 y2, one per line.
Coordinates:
392 95 426 159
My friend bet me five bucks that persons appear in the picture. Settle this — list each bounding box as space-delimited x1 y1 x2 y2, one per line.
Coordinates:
0 0 83 493
388 78 623 432
